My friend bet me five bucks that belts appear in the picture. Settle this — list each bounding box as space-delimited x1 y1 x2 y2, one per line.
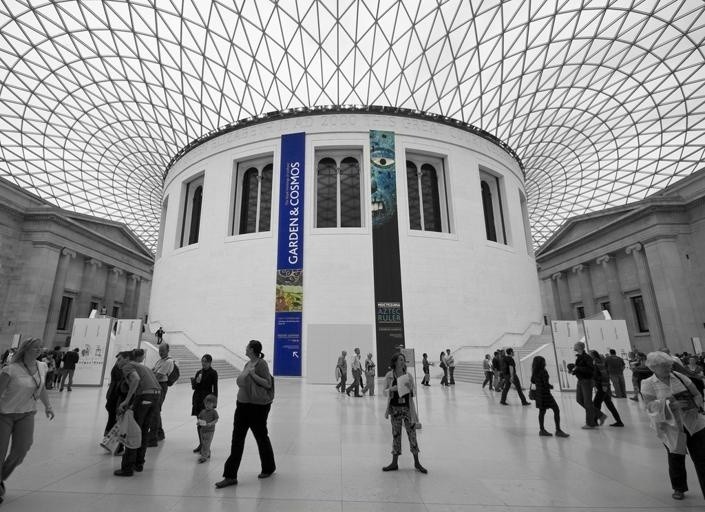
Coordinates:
140 389 161 394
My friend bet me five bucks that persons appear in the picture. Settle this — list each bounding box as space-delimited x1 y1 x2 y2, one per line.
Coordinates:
189 354 218 454
531 355 569 438
421 354 432 386
381 352 427 473
0 336 79 505
102 343 175 477
446 349 456 384
155 327 165 344
101 306 107 314
336 347 376 397
481 348 531 406
369 130 396 230
567 341 705 499
440 351 450 386
197 394 219 463
215 339 276 488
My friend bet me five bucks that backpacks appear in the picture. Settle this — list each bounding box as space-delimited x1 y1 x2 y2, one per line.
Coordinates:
167 357 180 386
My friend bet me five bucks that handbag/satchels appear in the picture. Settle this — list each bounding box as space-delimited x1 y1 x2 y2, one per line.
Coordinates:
246 373 274 404
530 390 537 399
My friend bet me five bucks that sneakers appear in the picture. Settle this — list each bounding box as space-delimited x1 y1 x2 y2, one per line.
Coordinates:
382 465 399 471
415 464 428 474
555 430 569 437
115 470 134 477
582 414 606 428
672 490 683 499
610 423 624 427
216 476 237 488
258 466 274 479
539 430 553 437
